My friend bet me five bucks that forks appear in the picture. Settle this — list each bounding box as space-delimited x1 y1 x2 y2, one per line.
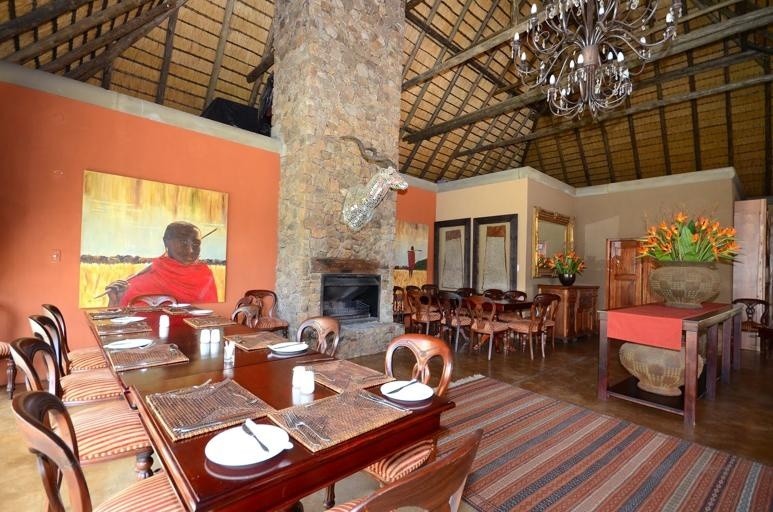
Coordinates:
281 409 330 448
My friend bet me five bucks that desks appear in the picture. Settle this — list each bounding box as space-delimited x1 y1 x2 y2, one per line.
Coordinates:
596 302 744 427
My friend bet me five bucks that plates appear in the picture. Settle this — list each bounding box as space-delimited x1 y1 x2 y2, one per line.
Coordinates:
102 337 152 349
267 341 308 354
203 424 290 469
107 303 213 323
204 457 284 481
379 380 433 403
265 352 307 360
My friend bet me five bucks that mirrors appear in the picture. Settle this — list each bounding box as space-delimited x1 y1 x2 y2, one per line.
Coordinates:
531 206 576 279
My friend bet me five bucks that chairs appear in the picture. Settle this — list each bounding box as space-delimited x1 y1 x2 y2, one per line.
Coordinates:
729 298 769 364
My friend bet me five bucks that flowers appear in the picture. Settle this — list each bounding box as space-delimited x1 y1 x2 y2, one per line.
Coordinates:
549 248 589 277
538 253 556 269
632 202 744 264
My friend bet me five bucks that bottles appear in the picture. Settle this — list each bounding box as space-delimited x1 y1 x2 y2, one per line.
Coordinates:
291 386 315 407
200 328 221 344
198 343 220 359
290 365 316 395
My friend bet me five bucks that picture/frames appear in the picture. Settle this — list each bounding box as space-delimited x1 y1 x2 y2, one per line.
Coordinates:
433 217 471 294
472 213 518 297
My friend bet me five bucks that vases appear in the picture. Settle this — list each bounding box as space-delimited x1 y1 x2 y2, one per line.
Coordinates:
559 274 575 286
646 261 722 310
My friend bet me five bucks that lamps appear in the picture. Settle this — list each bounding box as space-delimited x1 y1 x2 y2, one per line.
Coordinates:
510 0 683 121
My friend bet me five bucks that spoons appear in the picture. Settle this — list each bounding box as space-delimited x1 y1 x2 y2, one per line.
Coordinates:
168 343 181 356
228 391 258 405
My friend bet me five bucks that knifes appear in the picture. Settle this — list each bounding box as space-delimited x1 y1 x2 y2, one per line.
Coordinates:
359 392 409 413
241 422 269 452
386 380 419 393
171 411 256 433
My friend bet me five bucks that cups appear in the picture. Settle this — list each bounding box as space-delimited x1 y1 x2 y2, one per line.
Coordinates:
158 327 169 338
159 314 169 329
222 346 235 364
222 363 235 379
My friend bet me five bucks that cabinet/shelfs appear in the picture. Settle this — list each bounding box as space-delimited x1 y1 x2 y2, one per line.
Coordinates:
537 284 601 343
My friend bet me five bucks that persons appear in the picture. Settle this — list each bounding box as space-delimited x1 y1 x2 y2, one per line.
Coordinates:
407 246 416 278
104 221 219 307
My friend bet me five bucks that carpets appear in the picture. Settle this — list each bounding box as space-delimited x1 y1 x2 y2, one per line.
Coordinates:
433 372 772 512
